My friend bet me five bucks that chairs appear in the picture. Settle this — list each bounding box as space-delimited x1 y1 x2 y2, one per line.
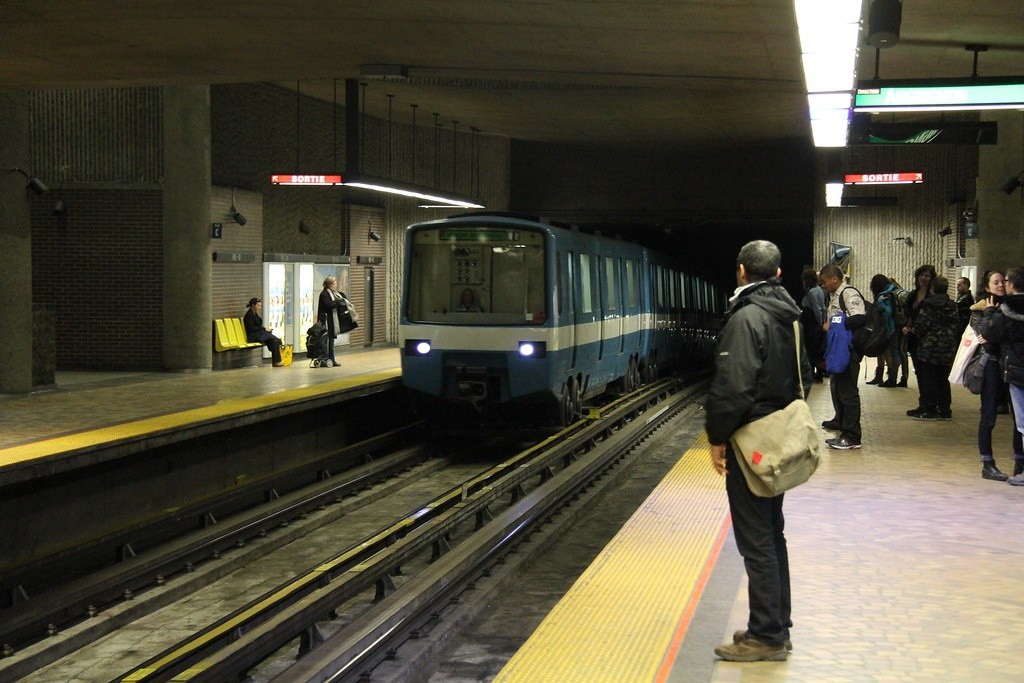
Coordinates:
214 319 246 353
222 318 253 348
232 318 261 348
241 317 266 347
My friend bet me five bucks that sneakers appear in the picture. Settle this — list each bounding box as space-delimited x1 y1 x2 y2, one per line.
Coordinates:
912 409 952 421
982 466 1009 481
1008 471 1024 486
1013 464 1024 476
826 434 861 450
714 630 792 661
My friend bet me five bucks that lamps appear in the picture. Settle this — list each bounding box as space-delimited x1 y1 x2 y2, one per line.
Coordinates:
939 223 953 237
13 168 48 197
53 199 64 216
299 222 312 236
233 213 247 226
790 0 1024 207
269 79 488 209
368 232 380 241
905 237 911 244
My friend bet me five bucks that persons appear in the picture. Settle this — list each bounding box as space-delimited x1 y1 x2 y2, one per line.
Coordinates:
318 276 346 366
243 298 285 367
704 240 812 662
800 263 1024 486
457 288 480 312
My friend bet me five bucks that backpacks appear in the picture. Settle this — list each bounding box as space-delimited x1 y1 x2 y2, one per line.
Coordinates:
306 321 330 362
826 287 891 356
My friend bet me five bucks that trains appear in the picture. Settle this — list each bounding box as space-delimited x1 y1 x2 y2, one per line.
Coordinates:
398 208 743 430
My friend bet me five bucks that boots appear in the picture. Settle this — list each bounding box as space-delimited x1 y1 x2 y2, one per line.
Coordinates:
877 370 896 387
897 376 907 387
866 367 883 384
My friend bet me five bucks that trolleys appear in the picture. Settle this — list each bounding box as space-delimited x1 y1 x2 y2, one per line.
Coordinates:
314 313 333 367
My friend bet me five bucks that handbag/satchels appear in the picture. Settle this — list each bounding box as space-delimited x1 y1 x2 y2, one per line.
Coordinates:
963 353 986 395
947 316 982 386
279 345 293 366
729 393 819 497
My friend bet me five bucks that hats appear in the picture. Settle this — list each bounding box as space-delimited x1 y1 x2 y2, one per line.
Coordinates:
249 298 261 304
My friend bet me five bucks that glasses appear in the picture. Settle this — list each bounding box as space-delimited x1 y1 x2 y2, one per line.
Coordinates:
1003 280 1009 284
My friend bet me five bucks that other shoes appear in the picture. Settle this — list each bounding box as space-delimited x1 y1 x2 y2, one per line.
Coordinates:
996 404 1010 415
822 416 840 429
334 361 341 366
907 406 924 416
